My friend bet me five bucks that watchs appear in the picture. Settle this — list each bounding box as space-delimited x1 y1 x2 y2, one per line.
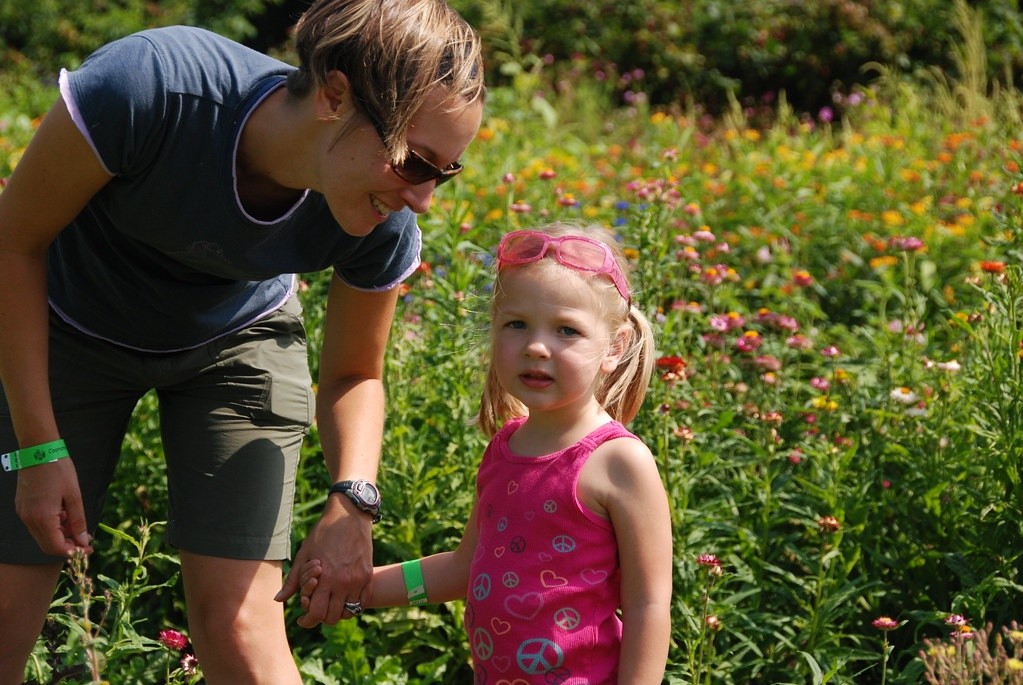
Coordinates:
327 479 383 524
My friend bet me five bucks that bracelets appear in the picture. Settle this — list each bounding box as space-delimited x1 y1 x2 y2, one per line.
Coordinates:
1 437 69 472
401 558 429 608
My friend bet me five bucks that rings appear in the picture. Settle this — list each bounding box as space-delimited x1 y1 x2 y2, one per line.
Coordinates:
344 600 363 615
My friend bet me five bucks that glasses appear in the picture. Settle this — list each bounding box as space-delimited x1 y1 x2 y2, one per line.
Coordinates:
350 85 464 189
497 232 630 305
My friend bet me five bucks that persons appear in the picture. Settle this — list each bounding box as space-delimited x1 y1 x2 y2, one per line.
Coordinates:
295 221 677 685
1 0 487 685
